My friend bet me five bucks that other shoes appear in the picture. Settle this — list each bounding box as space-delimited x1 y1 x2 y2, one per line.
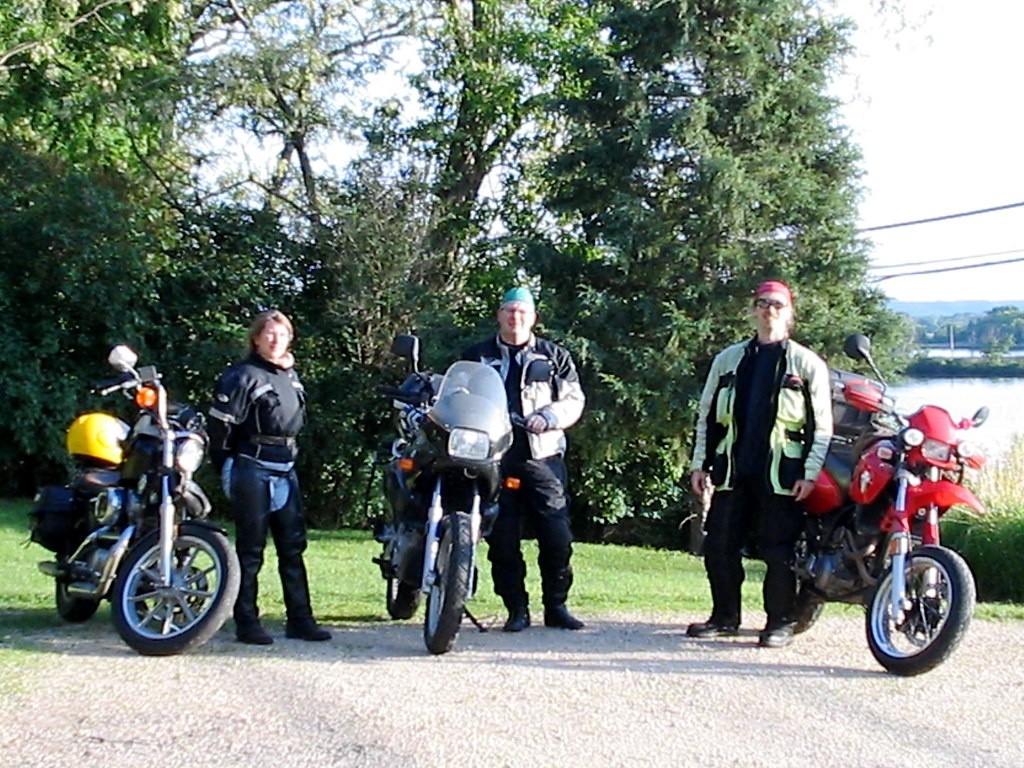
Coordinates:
285 620 331 642
544 604 583 630
504 606 530 632
685 612 740 638
236 626 274 645
759 613 798 647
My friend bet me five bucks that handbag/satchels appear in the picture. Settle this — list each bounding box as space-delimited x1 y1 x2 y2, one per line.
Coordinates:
30 486 89 553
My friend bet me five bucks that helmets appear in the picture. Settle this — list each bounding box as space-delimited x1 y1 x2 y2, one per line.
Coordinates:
67 412 130 467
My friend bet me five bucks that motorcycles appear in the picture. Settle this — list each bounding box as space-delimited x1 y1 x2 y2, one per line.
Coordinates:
27 345 242 655
787 334 990 676
371 334 542 655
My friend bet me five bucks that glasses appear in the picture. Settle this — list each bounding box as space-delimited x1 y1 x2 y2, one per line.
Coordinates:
754 299 789 309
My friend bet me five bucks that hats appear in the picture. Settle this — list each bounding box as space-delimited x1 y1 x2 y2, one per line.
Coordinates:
499 287 534 310
754 281 791 301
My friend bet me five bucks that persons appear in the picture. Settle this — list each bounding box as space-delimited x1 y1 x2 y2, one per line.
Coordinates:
461 286 587 630
206 310 333 644
685 280 833 646
